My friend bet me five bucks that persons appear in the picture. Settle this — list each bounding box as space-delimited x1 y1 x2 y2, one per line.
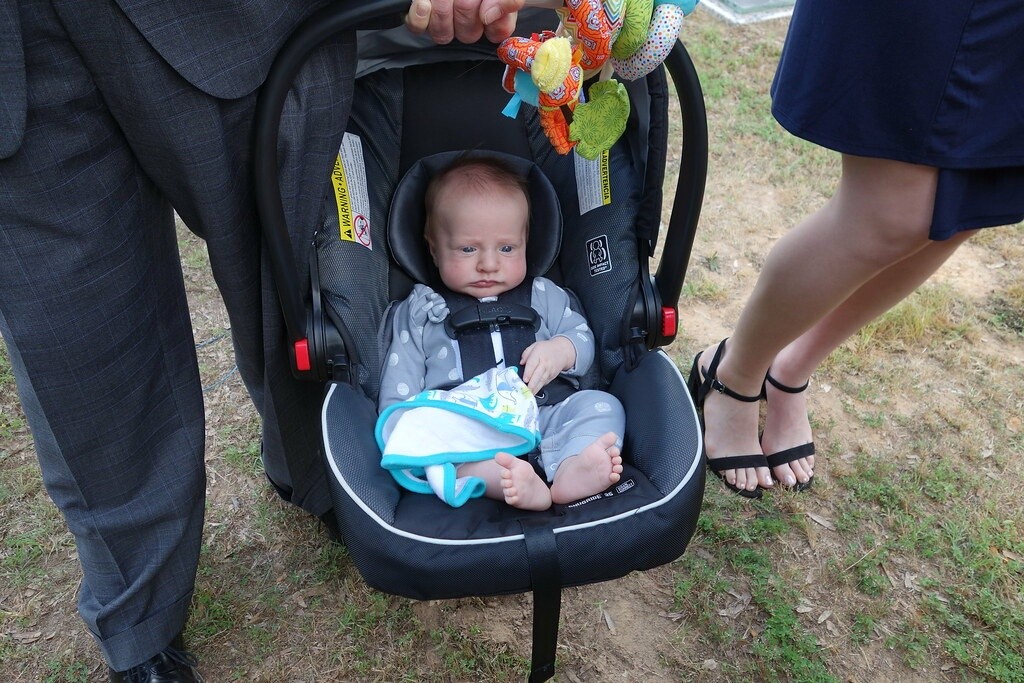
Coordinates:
374 158 626 512
0 1 523 683
689 0 1024 498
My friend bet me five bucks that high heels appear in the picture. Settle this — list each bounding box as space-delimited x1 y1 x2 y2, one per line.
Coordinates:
759 366 815 491
688 337 770 499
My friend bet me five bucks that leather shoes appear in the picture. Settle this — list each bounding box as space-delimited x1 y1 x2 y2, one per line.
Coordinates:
109 632 198 683
261 442 347 546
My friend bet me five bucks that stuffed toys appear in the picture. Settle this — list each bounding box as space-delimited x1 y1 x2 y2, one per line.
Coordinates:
499 0 696 158
